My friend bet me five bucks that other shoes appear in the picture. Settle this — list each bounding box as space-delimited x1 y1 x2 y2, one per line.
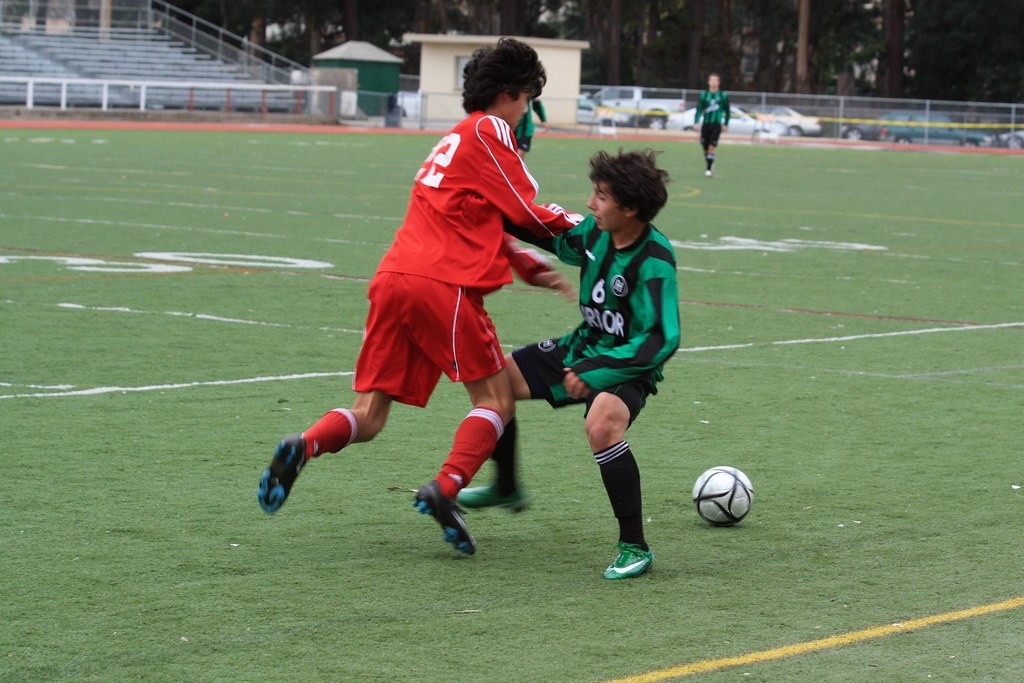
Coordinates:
705 170 713 177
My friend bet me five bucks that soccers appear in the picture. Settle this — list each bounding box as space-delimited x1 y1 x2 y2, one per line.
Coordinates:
691 465 755 527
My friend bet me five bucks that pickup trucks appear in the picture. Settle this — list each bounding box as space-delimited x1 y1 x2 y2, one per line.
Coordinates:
594 85 685 129
396 87 420 119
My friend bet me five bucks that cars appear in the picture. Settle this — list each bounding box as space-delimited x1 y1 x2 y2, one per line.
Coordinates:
666 104 787 137
879 111 993 148
578 95 630 127
841 114 883 141
998 127 1024 150
753 105 822 137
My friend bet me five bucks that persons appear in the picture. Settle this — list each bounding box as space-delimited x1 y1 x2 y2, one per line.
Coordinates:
514 97 549 158
458 149 681 580
258 36 585 556
693 73 730 177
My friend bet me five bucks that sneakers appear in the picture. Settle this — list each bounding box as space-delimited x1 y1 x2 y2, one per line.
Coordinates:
457 484 522 509
412 480 477 555
258 435 306 513
603 541 654 580
